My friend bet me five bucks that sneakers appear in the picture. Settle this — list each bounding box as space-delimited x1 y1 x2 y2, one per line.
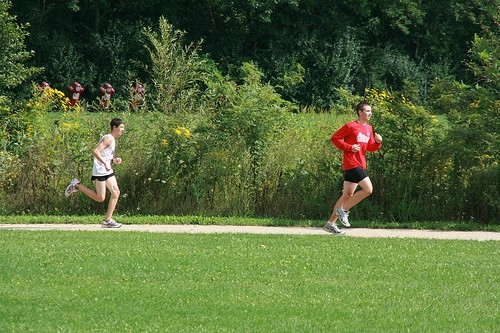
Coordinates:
322 221 345 236
338 206 351 227
101 219 122 228
64 178 80 198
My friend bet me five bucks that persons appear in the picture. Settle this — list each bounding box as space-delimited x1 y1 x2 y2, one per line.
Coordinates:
322 102 383 236
66 116 125 228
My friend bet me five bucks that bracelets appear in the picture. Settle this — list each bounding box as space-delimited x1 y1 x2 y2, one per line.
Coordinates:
111 159 114 163
